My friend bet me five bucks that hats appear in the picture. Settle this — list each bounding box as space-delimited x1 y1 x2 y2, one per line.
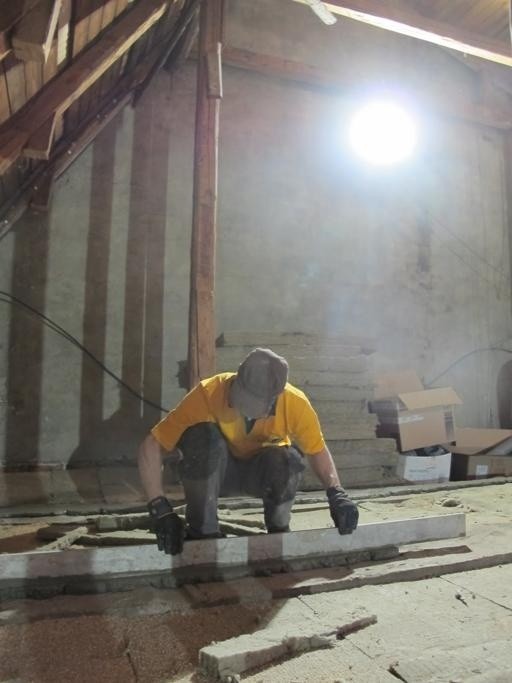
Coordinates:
232 348 288 418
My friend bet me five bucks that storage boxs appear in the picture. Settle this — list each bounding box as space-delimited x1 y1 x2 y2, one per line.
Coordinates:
453 427 512 479
391 446 452 481
368 368 462 451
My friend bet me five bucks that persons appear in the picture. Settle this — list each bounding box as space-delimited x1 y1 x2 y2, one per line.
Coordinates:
138 345 360 555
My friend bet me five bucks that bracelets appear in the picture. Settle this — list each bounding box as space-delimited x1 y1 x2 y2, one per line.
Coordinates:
149 497 173 515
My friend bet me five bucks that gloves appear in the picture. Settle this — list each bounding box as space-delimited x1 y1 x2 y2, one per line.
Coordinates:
327 486 358 534
148 497 185 555
